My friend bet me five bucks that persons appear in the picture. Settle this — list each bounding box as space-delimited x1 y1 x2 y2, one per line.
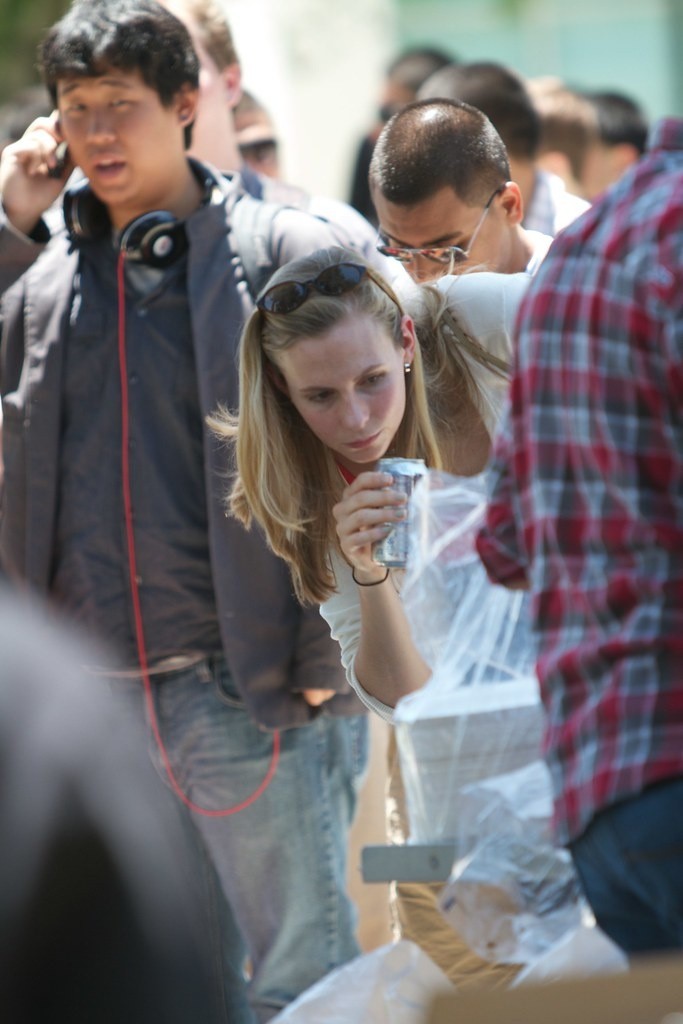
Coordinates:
473 113 683 974
218 236 543 860
364 99 561 284
155 1 652 264
0 0 393 1024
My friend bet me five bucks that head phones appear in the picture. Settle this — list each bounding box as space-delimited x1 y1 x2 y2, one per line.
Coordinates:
62 157 218 268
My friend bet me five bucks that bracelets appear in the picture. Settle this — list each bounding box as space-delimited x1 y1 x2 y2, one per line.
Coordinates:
350 566 389 588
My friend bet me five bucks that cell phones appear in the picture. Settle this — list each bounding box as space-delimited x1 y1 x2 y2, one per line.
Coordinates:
46 140 67 179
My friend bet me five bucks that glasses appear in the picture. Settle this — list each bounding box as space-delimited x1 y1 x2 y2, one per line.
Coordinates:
256 262 403 326
376 185 504 264
380 106 394 123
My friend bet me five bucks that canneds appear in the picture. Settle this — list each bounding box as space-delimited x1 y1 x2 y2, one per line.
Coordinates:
370 458 430 568
